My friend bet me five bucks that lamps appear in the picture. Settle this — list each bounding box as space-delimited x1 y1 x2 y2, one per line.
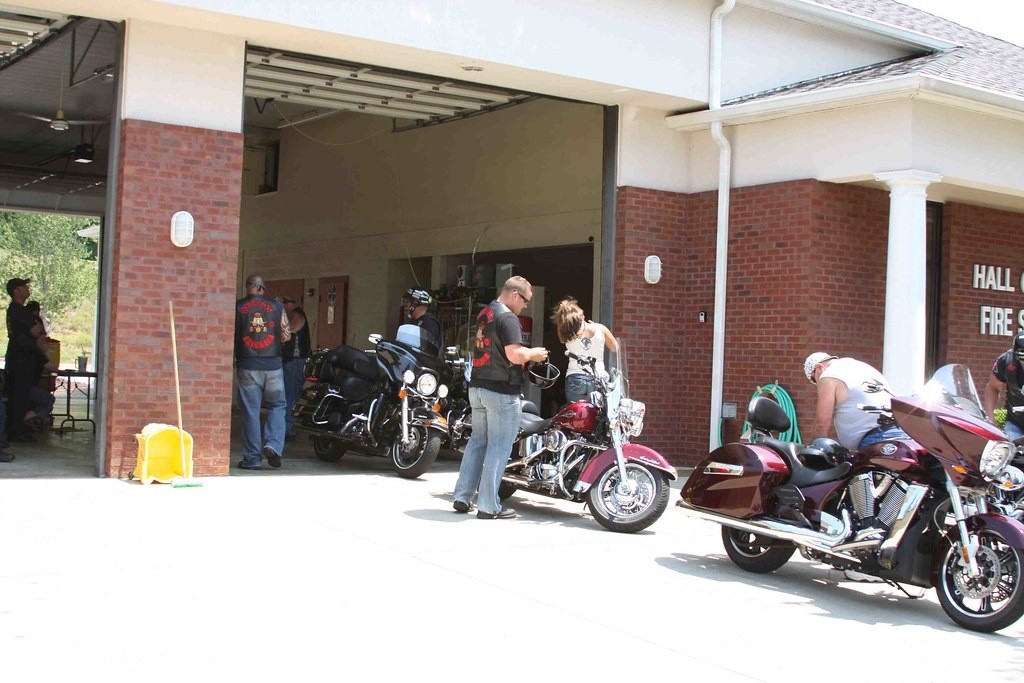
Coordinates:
73 144 94 165
644 255 664 285
170 210 195 248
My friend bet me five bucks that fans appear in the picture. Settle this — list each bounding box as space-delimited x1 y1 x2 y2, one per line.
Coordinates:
10 59 107 134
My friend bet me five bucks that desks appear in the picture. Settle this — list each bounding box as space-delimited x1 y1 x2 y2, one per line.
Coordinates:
50 369 97 439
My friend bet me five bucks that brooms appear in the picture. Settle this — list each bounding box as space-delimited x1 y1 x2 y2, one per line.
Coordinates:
168 300 204 488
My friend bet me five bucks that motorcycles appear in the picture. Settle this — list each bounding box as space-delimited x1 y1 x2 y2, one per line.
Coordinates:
449 336 678 534
289 324 479 479
944 416 1024 530
676 363 1024 633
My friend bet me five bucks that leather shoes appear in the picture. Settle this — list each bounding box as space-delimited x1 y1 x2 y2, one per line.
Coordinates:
477 506 516 518
6 432 37 443
454 500 476 512
0 443 15 462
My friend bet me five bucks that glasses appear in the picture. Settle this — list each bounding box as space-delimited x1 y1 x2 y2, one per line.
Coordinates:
514 290 528 304
259 285 265 290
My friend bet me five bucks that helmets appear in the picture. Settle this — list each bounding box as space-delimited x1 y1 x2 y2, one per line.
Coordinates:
527 362 560 389
1013 331 1024 360
798 437 844 469
401 286 433 305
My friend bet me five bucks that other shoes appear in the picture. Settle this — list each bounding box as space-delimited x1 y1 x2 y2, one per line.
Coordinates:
239 461 263 470
24 418 41 431
263 447 281 467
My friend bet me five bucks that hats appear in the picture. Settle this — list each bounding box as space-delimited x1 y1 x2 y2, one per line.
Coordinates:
26 301 39 311
804 350 838 385
7 278 31 296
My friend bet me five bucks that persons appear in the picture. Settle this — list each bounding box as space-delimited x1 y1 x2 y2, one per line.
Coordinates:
233 276 313 470
402 286 445 363
985 331 1024 441
0 278 56 462
804 351 920 582
452 276 547 519
551 299 621 405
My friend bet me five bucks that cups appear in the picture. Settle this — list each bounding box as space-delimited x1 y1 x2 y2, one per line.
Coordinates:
75 356 88 372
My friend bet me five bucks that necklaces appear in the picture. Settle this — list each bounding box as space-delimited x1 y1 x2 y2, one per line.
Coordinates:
576 322 586 337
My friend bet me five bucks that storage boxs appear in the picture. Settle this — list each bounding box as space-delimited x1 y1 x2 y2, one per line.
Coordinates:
46 342 60 368
303 352 332 384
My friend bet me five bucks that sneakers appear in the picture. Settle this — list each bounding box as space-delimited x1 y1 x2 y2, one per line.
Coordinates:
845 569 891 583
